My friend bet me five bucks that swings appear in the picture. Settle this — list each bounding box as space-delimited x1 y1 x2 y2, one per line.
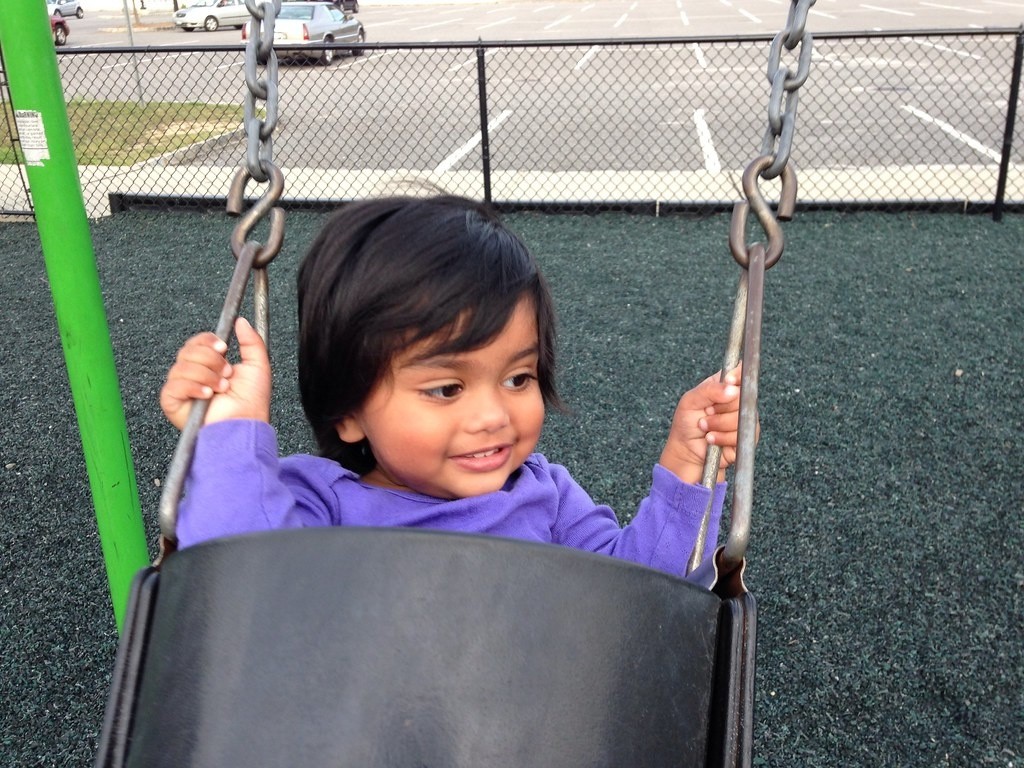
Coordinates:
90 0 816 768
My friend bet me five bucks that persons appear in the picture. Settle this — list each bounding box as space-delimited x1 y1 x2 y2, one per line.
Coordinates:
157 194 761 581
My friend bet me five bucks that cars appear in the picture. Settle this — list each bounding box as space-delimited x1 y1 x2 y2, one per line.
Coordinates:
49 15 71 46
172 0 272 31
304 0 360 14
46 0 84 19
241 1 367 65
